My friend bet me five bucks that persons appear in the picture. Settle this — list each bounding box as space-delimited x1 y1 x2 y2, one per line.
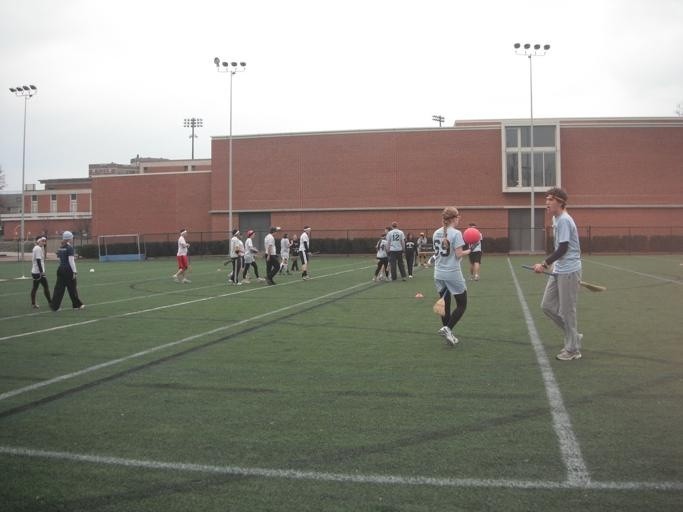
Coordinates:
52 231 86 311
533 187 584 361
173 229 191 283
372 222 428 282
228 225 312 286
432 207 480 345
30 235 52 309
40 231 48 260
468 223 483 280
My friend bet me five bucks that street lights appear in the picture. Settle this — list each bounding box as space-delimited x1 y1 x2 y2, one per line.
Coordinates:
214 57 246 260
432 115 445 127
182 117 202 160
513 42 549 252
8 84 36 281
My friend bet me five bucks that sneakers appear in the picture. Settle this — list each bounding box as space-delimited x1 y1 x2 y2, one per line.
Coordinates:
172 275 191 284
227 273 276 288
556 334 584 361
438 326 459 346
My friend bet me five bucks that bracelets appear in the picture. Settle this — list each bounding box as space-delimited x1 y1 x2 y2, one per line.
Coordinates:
541 262 547 268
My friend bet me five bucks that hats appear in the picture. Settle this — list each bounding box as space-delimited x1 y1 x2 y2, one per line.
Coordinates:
63 231 73 240
271 227 280 232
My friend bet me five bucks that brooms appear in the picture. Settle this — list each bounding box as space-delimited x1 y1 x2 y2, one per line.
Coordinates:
521 265 606 293
432 287 449 316
